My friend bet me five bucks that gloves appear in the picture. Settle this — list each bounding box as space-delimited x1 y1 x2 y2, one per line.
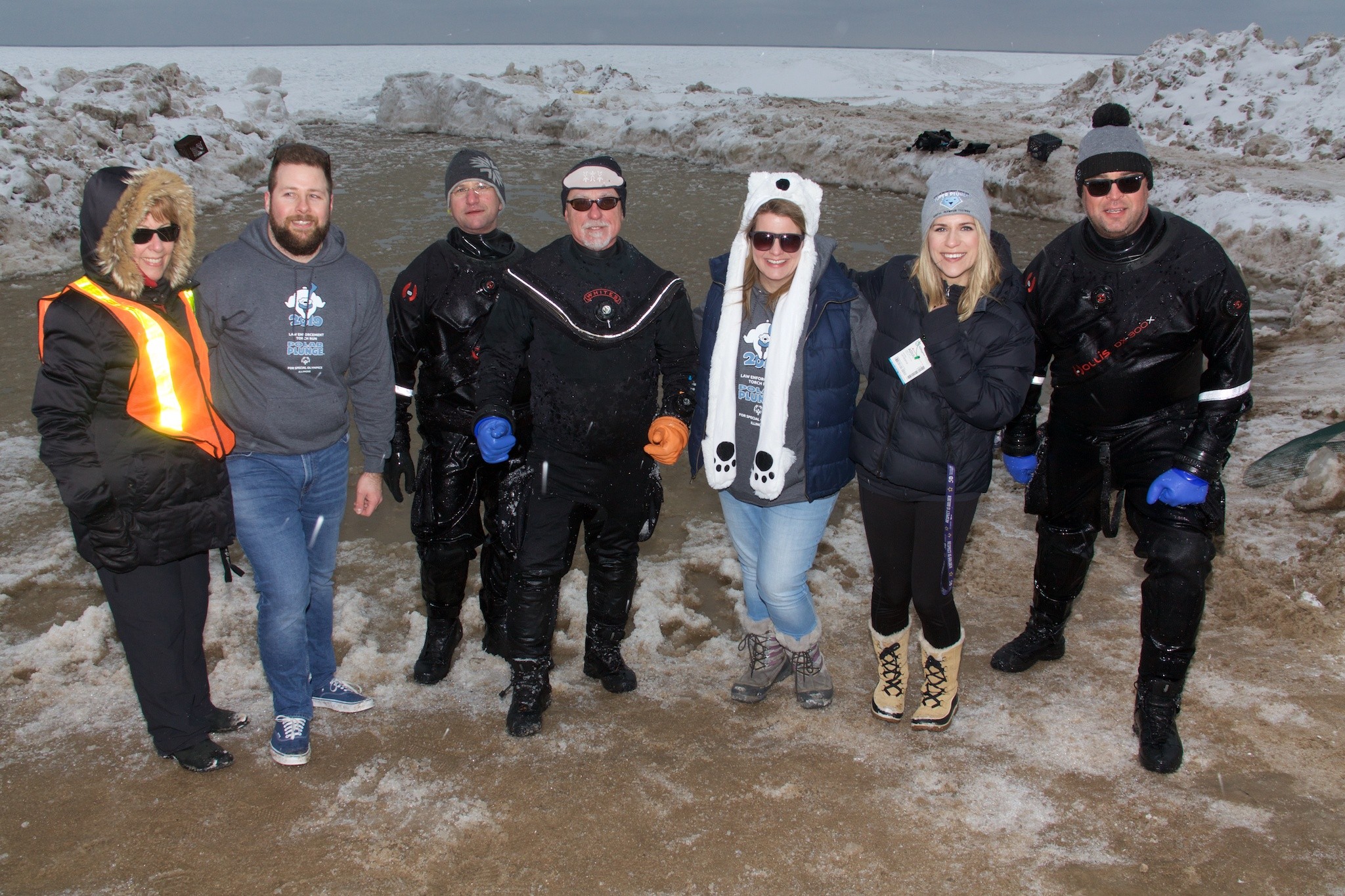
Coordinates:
1146 468 1210 507
474 417 514 463
82 501 151 574
1002 453 1037 484
643 416 689 466
383 443 417 504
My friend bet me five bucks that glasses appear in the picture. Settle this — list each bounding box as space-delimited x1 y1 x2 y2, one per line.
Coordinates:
1083 173 1147 197
566 196 621 211
132 223 180 244
749 231 803 254
451 183 494 197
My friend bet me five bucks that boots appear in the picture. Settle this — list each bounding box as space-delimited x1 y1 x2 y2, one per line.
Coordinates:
774 615 834 709
731 616 793 703
910 627 965 732
868 615 911 724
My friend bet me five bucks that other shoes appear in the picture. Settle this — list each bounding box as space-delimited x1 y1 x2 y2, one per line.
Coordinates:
167 706 249 772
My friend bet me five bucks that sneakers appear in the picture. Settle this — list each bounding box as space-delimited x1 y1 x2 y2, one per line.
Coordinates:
990 606 1064 673
1132 675 1183 774
270 677 375 764
413 616 637 733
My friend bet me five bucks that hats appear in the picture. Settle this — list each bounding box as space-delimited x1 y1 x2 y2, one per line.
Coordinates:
702 171 823 501
921 156 992 243
561 157 627 218
1074 103 1153 198
445 149 506 215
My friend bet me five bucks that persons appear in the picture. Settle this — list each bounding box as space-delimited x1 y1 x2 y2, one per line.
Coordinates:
687 171 877 708
836 156 1037 733
29 167 250 771
474 156 698 739
382 149 534 688
191 143 395 766
990 102 1255 775
